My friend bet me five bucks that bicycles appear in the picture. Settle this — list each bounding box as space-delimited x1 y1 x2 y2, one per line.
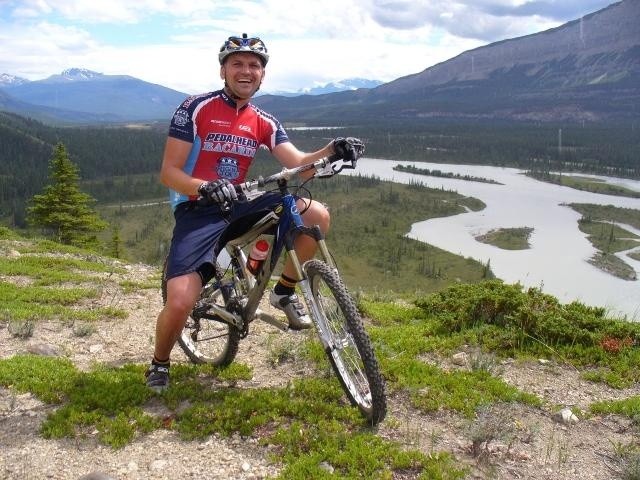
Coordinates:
161 153 388 426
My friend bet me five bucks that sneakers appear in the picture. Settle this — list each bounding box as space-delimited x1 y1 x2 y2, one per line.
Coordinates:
268 288 314 330
144 364 171 396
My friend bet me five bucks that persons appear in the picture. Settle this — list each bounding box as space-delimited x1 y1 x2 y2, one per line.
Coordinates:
144 33 364 390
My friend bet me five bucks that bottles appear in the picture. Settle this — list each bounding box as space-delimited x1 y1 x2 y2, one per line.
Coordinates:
246 239 270 276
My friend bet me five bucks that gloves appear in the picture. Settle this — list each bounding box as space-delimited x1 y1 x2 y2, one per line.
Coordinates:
197 178 238 205
329 136 366 169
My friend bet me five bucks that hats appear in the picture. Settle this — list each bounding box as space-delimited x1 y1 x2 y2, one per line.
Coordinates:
217 32 270 68
